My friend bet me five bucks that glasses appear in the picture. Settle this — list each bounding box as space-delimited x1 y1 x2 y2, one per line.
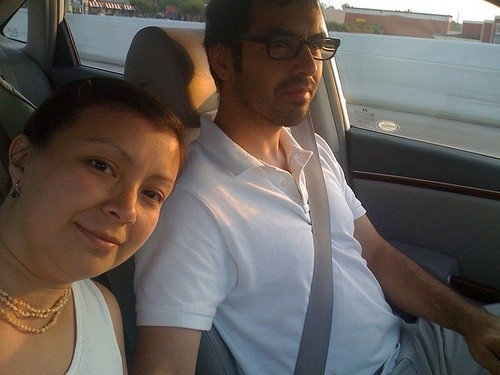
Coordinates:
234 32 341 62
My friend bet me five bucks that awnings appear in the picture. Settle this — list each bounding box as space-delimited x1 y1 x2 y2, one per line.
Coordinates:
89 1 135 11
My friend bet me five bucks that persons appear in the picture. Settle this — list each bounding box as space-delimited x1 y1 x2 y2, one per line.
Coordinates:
132 0 500 375
0 76 188 375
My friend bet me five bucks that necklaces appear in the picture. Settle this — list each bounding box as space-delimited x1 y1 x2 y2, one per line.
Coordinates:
0 287 72 333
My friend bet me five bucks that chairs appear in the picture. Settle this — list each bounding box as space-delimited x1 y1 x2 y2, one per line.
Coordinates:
0 27 238 375
